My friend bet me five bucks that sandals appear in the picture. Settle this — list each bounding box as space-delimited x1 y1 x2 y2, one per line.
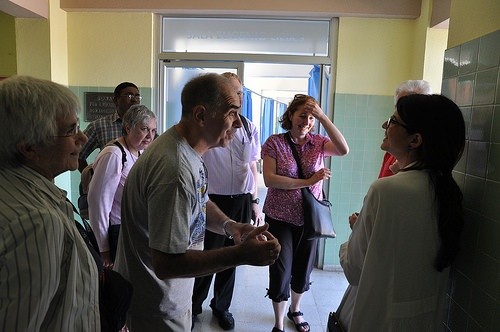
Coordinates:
287 305 310 332
272 326 285 332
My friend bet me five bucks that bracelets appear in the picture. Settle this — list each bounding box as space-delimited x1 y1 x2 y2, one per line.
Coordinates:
223 220 237 239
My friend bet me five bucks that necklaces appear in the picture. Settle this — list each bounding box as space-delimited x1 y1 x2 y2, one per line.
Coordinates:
123 136 135 162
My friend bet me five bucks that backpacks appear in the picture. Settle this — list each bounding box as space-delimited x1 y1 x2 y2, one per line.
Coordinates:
78 141 127 220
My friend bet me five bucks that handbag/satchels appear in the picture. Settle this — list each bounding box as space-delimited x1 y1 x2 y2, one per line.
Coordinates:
304 190 337 240
327 311 346 332
107 224 122 262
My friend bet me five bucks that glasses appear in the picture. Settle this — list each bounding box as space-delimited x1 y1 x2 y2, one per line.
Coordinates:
122 95 143 102
293 94 306 99
53 119 81 138
387 117 407 129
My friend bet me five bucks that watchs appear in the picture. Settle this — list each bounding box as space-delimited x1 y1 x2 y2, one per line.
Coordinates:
252 198 260 204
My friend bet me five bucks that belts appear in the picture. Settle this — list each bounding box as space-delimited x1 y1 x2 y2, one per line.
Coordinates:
209 192 249 201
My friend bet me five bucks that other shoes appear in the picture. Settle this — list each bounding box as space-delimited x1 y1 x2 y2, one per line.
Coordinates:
210 304 235 331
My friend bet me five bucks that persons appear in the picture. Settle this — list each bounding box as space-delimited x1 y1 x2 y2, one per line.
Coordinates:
0 76 101 332
78 82 142 174
191 72 261 331
110 73 281 332
261 94 349 332
335 80 470 332
87 105 157 332
